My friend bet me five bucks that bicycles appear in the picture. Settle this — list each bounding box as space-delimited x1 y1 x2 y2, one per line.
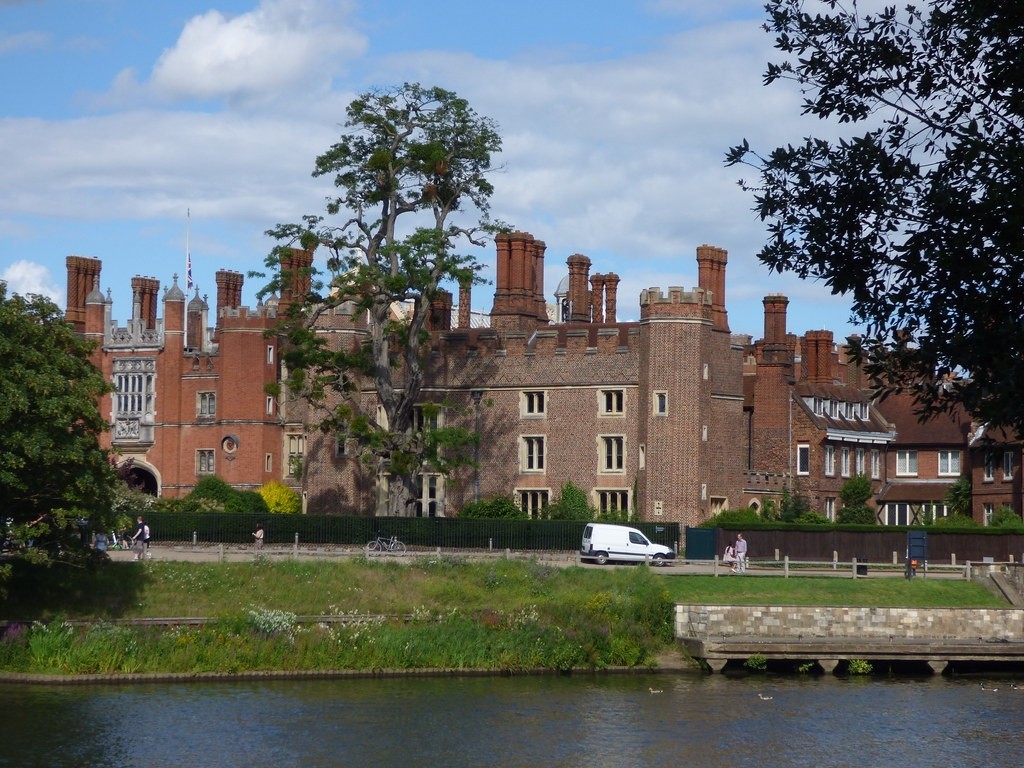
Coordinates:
368 531 407 558
102 534 135 551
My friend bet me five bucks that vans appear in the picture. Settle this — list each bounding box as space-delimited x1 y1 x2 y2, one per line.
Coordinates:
580 522 675 567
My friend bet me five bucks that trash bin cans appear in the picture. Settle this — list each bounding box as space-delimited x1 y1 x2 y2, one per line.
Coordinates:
857 559 868 575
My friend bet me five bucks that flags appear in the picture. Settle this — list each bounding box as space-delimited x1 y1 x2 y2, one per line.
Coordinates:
188 254 193 289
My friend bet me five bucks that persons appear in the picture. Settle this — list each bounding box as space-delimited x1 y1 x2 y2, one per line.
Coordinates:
735 534 747 573
93 515 152 560
723 541 737 572
252 521 263 558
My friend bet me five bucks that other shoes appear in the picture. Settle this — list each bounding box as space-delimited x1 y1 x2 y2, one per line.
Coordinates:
148 553 152 559
134 556 138 559
731 569 737 573
